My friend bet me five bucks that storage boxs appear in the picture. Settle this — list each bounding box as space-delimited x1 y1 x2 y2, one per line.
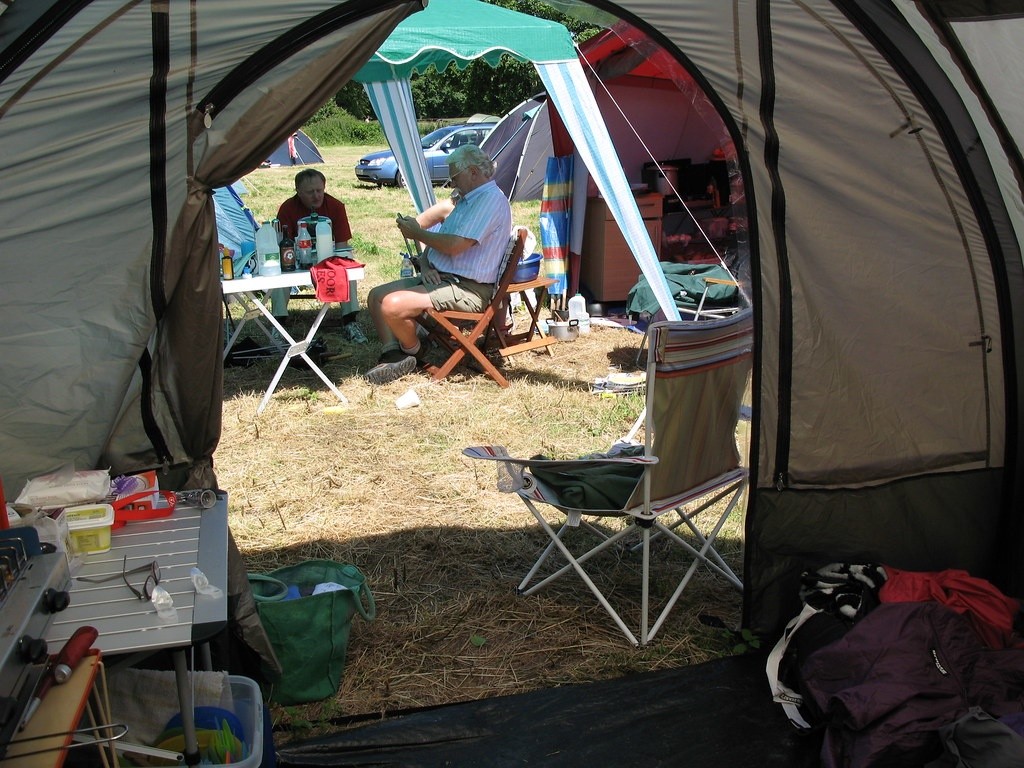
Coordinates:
34 506 75 565
64 503 115 558
116 471 160 510
158 674 264 768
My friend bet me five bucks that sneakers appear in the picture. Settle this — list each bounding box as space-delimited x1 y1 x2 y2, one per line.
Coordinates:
269 327 286 346
399 338 432 360
364 349 416 385
343 322 368 344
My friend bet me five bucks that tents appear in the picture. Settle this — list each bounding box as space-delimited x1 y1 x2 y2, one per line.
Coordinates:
211 180 300 295
545 19 746 310
260 128 325 166
450 91 555 204
350 0 683 321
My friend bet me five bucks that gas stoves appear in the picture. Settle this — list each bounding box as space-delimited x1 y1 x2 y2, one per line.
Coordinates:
0 527 71 757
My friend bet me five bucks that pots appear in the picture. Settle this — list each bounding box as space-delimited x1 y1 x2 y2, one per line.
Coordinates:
546 317 579 342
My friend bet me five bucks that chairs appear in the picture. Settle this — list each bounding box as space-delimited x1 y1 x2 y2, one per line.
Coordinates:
635 276 745 371
416 227 529 389
461 307 755 649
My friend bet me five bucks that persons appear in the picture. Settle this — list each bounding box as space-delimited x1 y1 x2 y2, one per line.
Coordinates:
362 144 512 387
364 116 370 123
270 168 368 347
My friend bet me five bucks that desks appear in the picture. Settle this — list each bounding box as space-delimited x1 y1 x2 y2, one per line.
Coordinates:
0 648 120 768
491 276 560 368
41 491 229 768
221 264 366 414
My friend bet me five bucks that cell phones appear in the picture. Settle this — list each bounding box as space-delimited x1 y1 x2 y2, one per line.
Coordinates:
397 213 403 220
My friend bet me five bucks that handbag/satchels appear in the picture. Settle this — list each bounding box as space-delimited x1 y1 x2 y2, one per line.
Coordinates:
235 560 376 706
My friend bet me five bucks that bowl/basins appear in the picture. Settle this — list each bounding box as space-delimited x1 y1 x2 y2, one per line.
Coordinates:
508 253 543 284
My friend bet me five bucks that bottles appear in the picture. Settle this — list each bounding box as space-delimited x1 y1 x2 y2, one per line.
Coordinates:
221 247 234 279
568 292 588 336
255 212 334 277
400 254 414 279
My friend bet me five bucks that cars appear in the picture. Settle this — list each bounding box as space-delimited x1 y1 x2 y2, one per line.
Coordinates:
355 121 497 188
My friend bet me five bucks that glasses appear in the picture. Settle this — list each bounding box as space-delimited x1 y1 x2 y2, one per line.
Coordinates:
450 169 464 181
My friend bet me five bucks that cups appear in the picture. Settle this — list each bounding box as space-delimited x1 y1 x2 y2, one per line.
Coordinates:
396 389 420 411
176 490 216 508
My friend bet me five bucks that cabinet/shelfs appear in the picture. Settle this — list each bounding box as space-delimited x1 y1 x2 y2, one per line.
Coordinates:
580 193 663 317
